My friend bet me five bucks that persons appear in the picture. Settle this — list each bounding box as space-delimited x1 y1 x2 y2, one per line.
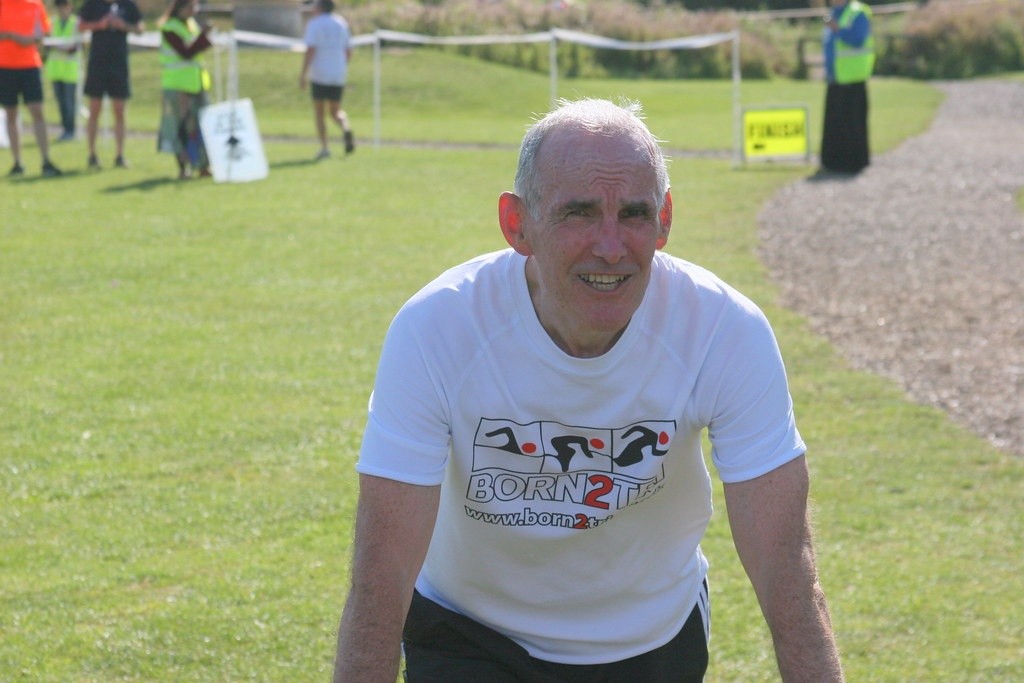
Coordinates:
73 0 145 171
327 93 844 682
0 0 62 178
806 1 874 183
44 0 84 141
157 0 216 179
299 0 356 161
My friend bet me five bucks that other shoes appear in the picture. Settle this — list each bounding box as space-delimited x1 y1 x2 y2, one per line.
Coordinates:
40 161 62 176
9 162 25 176
177 169 192 180
115 156 130 169
807 167 855 182
316 149 332 162
88 156 102 170
344 132 354 153
